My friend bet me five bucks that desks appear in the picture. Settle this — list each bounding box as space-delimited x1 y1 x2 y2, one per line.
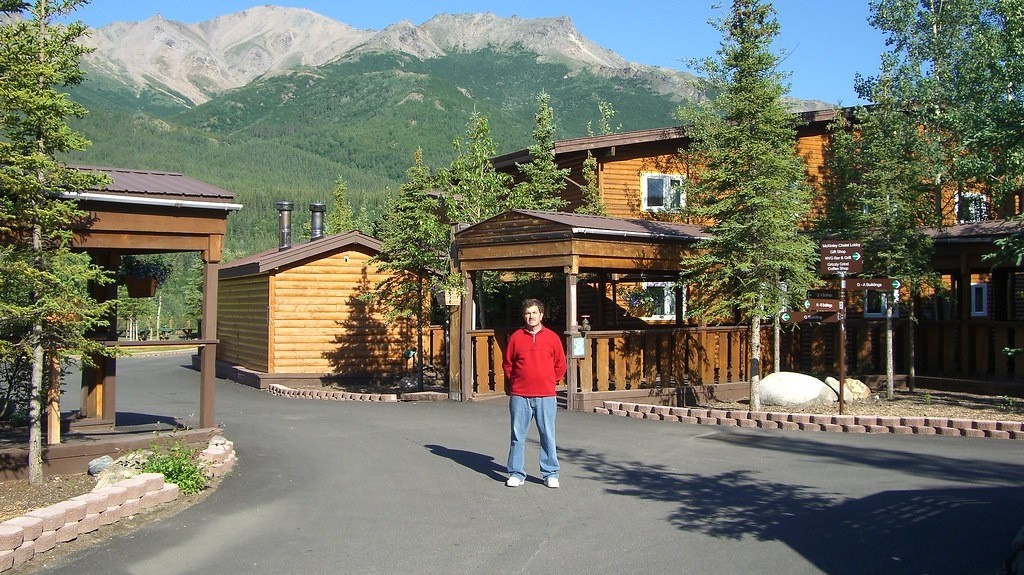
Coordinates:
160 328 173 340
181 326 194 340
138 330 151 341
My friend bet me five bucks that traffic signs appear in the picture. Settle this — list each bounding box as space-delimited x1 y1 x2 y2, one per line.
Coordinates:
802 298 841 313
820 239 864 274
777 311 841 323
844 278 903 291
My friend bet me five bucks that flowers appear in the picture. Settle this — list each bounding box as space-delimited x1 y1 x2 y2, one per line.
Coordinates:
430 270 471 307
618 285 664 317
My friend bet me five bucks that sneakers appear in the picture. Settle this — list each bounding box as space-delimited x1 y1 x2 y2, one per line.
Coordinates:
507 476 524 487
544 478 559 488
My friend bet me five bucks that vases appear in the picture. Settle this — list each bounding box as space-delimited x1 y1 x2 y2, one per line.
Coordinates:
436 287 461 306
630 305 653 317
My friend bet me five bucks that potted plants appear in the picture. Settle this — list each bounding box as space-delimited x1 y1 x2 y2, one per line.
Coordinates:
116 252 174 298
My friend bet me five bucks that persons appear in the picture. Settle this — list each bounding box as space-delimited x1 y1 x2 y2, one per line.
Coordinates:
501 299 567 488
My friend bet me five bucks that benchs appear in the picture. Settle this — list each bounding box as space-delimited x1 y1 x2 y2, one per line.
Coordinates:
179 334 193 338
